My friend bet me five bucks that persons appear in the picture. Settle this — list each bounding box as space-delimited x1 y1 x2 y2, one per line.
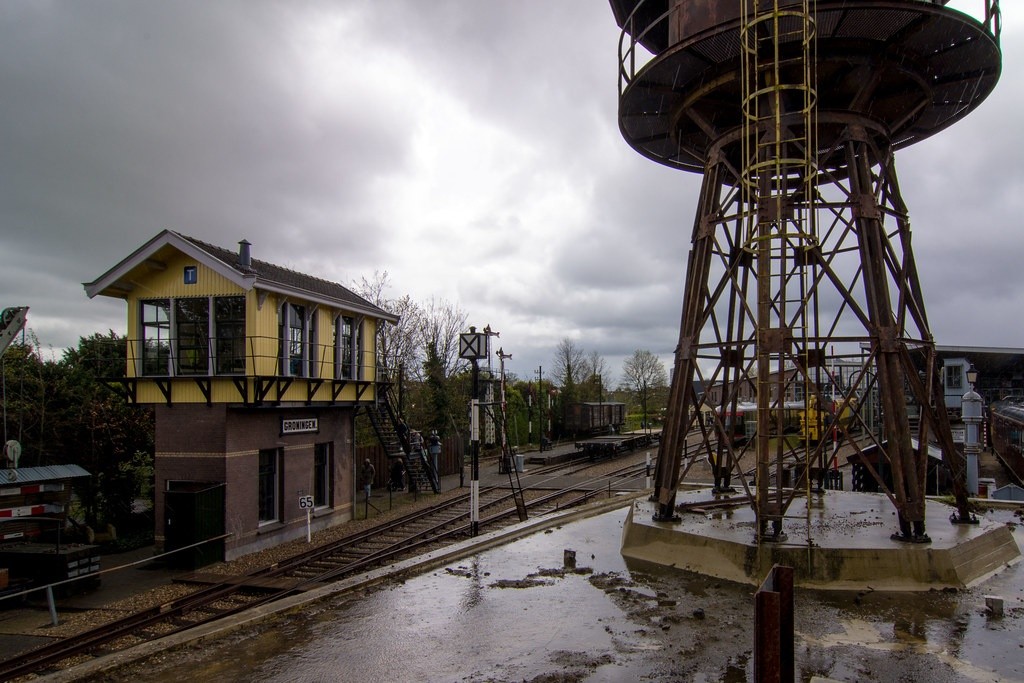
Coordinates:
377 390 429 467
391 458 406 492
361 459 375 500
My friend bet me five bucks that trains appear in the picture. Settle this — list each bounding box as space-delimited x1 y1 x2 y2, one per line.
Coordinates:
714 400 803 448
797 392 876 446
989 392 1024 487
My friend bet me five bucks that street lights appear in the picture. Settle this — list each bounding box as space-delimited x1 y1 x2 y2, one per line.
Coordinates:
960 363 984 498
